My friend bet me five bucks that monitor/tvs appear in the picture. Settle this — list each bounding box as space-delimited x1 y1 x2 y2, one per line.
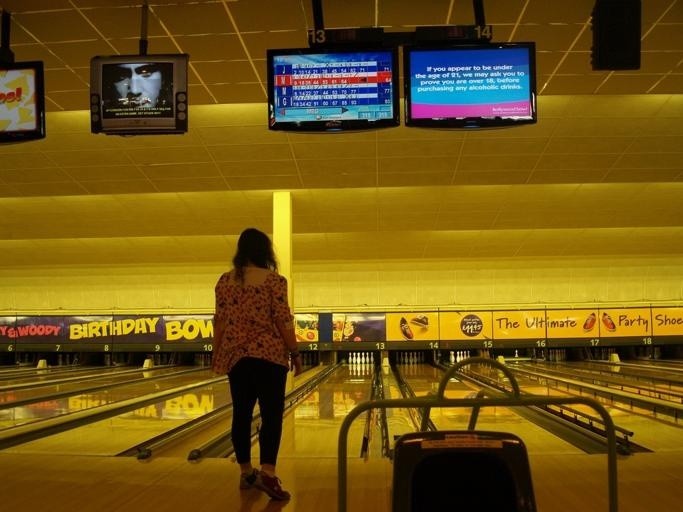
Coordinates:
0 60 46 143
266 43 400 133
87 54 190 135
401 42 537 129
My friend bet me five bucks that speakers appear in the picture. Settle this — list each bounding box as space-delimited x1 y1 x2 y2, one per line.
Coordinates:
590 0 641 69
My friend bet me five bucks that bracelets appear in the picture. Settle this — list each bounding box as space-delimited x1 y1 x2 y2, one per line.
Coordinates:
290 352 300 358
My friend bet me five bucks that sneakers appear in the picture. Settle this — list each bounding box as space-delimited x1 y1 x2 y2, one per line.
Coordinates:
239 468 258 490
253 470 291 500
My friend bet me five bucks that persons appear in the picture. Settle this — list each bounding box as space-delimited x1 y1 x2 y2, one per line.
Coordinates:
100 63 169 114
210 228 303 499
